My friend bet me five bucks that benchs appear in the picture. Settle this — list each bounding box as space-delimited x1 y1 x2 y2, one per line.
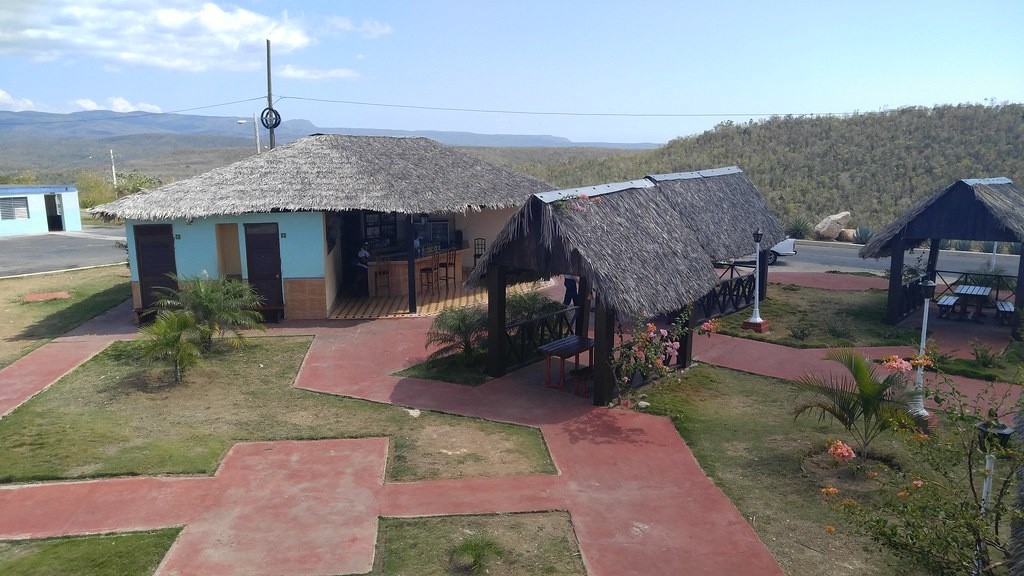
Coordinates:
570 365 594 398
936 295 960 320
994 301 1014 327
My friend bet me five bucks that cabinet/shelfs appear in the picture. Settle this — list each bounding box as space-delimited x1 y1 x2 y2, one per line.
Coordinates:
360 210 398 249
414 221 451 245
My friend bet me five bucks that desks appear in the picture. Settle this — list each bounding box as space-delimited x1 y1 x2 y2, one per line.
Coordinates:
537 335 595 393
954 284 993 325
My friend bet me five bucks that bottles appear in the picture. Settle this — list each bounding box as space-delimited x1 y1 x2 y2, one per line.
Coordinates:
420 242 441 252
368 238 390 248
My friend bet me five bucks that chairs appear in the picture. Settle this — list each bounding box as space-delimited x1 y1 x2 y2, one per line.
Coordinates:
419 251 440 295
374 255 390 298
473 237 487 267
439 248 457 290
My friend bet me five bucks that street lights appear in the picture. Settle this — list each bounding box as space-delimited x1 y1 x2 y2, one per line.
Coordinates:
974 408 1015 575
741 228 769 334
236 113 262 155
906 275 937 431
